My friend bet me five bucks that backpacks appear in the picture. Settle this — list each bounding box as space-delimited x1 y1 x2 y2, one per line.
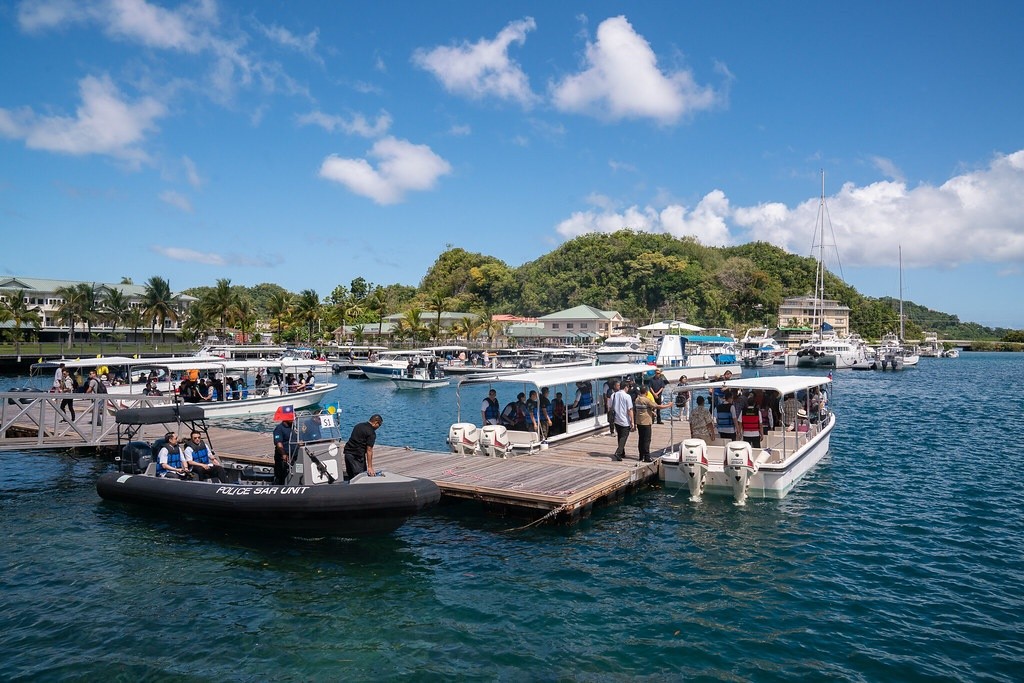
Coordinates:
91 378 107 394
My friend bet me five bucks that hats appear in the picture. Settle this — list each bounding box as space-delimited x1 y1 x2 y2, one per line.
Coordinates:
306 371 312 374
150 383 157 389
206 379 212 383
101 375 107 380
797 409 807 417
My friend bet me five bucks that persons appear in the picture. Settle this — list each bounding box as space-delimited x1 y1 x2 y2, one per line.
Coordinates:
458 350 489 367
138 369 178 396
690 370 826 448
678 375 690 420
86 370 101 426
407 358 439 379
273 410 322 484
481 387 564 440
53 364 75 423
649 370 665 425
371 351 378 363
603 378 673 462
255 371 315 393
101 372 126 386
350 348 355 364
178 374 248 403
156 431 229 483
573 381 593 419
343 414 383 478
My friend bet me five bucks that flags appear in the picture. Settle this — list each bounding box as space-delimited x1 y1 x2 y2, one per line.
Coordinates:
273 405 294 420
324 402 338 414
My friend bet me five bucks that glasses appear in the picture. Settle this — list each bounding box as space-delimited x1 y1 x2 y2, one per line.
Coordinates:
377 422 381 427
193 437 200 440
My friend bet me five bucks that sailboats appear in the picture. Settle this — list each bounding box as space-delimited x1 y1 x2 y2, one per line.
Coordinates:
879 245 922 365
797 169 875 369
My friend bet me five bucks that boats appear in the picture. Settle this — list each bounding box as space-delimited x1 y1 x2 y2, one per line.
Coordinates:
739 328 782 355
595 323 647 363
651 334 742 381
740 348 775 368
97 381 441 540
657 375 837 500
874 346 904 370
919 341 944 358
10 356 340 416
392 368 450 389
944 349 959 359
194 343 595 380
691 341 737 364
445 363 660 456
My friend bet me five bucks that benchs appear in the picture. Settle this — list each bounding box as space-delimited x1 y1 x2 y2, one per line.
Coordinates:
679 420 822 464
145 455 223 483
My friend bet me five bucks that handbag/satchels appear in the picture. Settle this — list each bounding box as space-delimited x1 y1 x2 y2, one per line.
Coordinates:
676 395 686 407
608 410 615 424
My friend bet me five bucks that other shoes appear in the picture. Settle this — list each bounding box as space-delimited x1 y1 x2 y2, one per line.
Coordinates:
657 421 664 424
614 453 622 461
639 457 643 460
645 457 656 463
622 454 625 457
610 431 616 437
59 419 69 425
